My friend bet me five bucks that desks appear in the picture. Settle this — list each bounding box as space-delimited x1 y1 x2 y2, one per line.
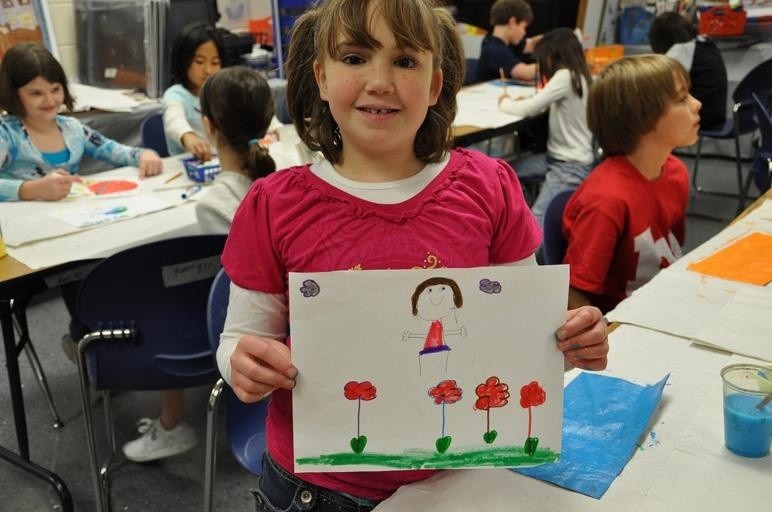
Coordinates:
367 185 772 511
1 120 324 512
446 71 559 151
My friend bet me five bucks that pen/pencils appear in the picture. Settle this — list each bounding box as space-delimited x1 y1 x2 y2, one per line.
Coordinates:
165 171 183 184
499 67 508 96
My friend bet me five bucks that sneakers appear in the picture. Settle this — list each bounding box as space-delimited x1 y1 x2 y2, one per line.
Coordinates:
123 418 196 462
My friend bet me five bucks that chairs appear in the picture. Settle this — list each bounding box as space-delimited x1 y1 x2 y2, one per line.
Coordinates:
685 95 759 225
752 88 772 195
142 113 171 158
541 186 577 264
65 234 241 512
203 260 277 512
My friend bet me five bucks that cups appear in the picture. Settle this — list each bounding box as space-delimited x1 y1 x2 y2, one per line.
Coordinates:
720 365 771 459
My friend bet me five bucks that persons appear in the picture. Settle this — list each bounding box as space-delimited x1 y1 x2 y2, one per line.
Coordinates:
1 40 165 202
160 20 281 163
498 26 607 253
648 11 727 132
560 53 705 317
472 0 547 83
194 65 277 236
213 0 611 512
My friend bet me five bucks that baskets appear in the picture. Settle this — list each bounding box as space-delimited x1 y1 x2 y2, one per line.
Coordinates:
182 154 221 184
